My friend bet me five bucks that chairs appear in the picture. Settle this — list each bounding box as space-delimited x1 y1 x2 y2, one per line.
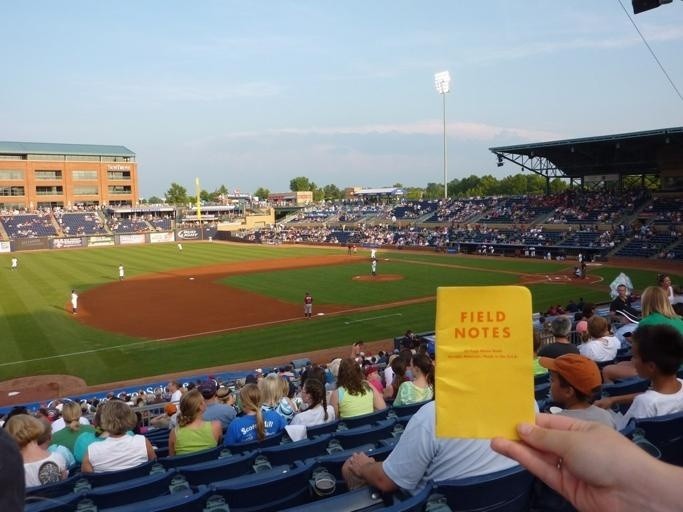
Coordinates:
21 372 681 510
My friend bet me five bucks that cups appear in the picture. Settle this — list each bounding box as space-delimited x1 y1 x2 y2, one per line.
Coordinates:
316 478 335 493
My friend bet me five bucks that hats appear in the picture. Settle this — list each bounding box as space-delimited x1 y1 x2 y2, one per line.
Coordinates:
576 321 587 333
198 378 219 400
164 403 177 414
538 353 602 396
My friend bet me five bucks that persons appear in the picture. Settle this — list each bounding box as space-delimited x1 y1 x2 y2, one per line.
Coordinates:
71 289 79 315
574 265 582 278
580 262 587 279
0 328 437 512
370 259 378 274
10 256 19 271
208 235 213 248
235 184 683 266
117 263 125 281
176 242 184 255
0 202 173 249
434 271 682 512
303 292 314 320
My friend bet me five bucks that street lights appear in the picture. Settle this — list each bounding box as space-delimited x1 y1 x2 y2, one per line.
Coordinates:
433 71 451 200
